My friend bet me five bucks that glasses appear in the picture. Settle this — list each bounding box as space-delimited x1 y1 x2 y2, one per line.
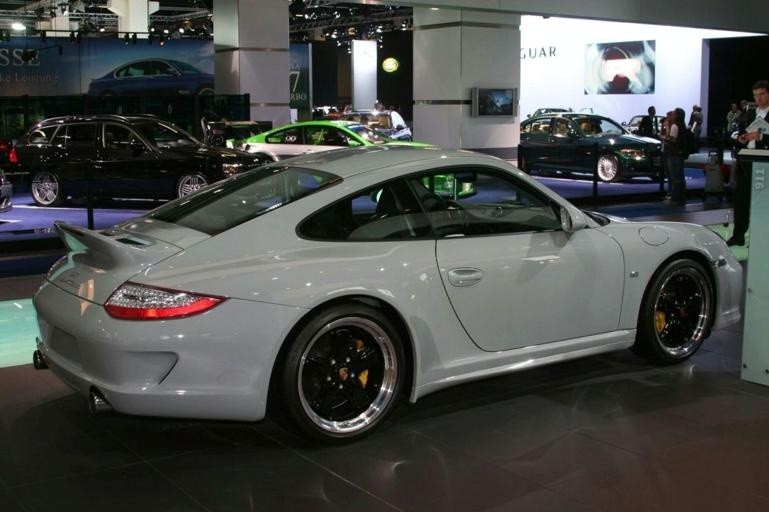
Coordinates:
753 93 765 98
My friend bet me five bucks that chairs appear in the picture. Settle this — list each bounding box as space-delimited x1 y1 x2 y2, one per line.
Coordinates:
106 132 115 159
373 179 446 238
533 122 596 136
123 68 157 76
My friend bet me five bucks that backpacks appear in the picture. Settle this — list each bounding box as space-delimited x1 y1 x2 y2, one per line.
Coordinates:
674 123 696 160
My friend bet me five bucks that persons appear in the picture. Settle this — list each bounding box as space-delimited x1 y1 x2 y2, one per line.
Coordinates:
339 99 394 129
723 80 769 247
663 107 689 210
661 111 675 200
692 106 703 153
637 106 660 140
688 104 699 130
734 99 748 135
726 103 742 152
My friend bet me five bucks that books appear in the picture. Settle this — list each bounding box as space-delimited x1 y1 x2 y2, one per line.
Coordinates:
745 118 769 135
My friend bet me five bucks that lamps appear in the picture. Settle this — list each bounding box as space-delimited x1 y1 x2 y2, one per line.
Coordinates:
295 0 409 53
22 0 184 62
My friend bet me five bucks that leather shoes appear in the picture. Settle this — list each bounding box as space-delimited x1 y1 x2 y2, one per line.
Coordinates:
726 234 745 246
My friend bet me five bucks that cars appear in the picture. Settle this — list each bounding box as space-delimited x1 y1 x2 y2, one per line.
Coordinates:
0 113 274 208
85 58 214 100
517 108 693 183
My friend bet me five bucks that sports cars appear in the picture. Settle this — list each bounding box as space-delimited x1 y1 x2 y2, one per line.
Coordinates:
32 145 744 446
199 116 476 200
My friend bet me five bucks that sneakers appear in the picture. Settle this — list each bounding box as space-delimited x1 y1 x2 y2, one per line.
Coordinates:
662 196 685 206
